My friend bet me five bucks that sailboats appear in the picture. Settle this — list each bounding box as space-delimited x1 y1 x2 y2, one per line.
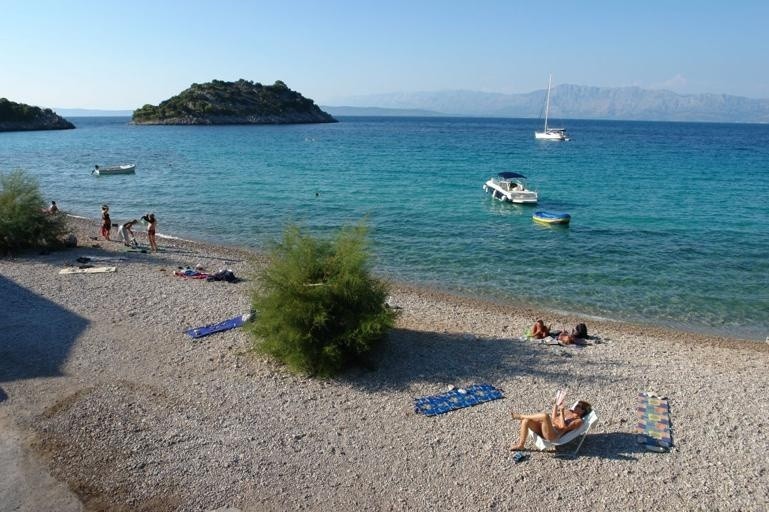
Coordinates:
534 73 567 141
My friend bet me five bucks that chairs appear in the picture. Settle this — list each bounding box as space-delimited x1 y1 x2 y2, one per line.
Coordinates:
528 411 598 457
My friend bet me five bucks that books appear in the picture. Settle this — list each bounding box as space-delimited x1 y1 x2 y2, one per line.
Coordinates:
556 390 566 405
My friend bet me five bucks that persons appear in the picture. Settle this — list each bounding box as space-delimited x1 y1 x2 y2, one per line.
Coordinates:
118 220 138 247
101 206 111 241
511 401 591 450
532 319 552 339
48 201 60 215
141 214 158 251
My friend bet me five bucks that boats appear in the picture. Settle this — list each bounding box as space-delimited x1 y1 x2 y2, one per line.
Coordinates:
90 163 136 175
532 211 571 224
482 172 538 204
